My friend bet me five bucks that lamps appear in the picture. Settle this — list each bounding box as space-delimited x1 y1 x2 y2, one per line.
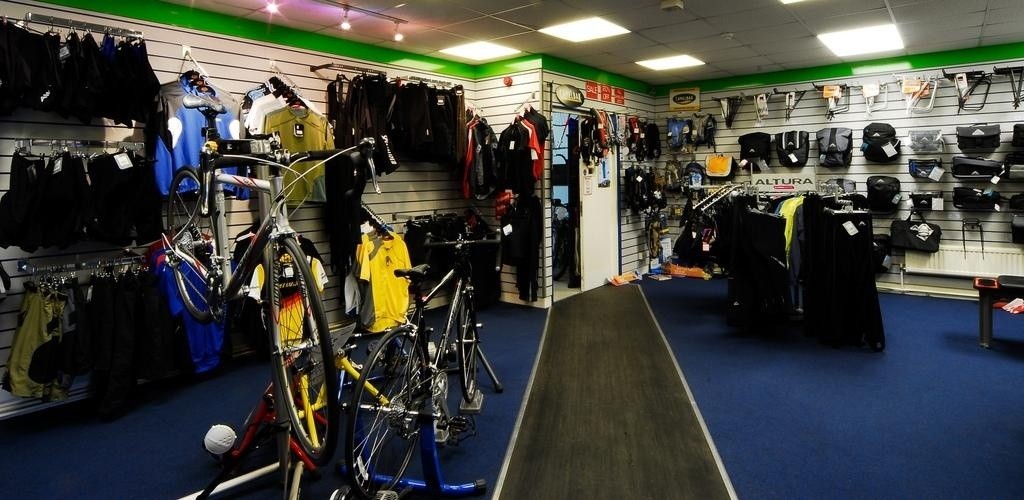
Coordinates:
313 0 409 43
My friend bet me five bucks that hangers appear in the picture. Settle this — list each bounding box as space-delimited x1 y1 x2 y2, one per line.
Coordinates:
180 58 309 110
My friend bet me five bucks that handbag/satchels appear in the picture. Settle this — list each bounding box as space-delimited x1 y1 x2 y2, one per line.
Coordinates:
863 124 900 162
956 125 1000 149
826 179 855 193
888 220 940 252
739 133 770 171
705 154 735 181
867 176 900 210
908 131 942 151
909 159 941 178
874 234 890 270
817 128 852 167
912 192 942 208
776 131 809 167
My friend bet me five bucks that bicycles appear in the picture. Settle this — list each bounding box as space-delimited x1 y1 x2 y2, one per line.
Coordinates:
346 231 504 498
163 94 381 468
552 198 572 280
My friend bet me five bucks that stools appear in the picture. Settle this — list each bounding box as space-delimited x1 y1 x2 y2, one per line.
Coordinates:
973 275 1024 349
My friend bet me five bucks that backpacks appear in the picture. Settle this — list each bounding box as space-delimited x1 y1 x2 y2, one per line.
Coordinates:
665 161 682 191
667 118 690 149
684 163 704 197
629 119 660 161
691 115 713 145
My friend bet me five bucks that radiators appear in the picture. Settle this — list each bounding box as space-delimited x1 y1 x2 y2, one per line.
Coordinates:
904 245 1024 278
